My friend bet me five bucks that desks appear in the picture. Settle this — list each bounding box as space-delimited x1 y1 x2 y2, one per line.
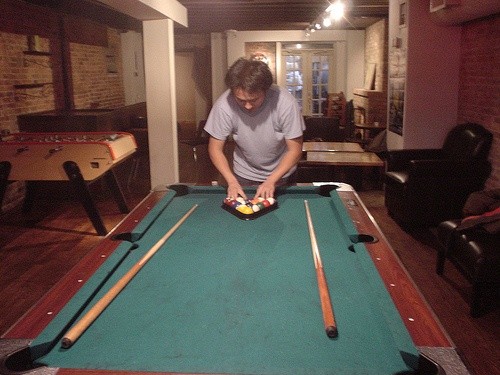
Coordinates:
355 124 385 149
302 142 365 153
0 181 471 375
307 152 382 166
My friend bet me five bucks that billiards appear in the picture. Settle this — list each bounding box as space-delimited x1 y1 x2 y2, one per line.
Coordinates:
256 202 264 210
267 198 275 205
261 200 270 208
252 204 260 212
224 196 275 215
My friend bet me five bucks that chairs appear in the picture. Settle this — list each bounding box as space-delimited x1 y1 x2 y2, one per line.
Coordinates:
436 189 500 317
181 119 209 179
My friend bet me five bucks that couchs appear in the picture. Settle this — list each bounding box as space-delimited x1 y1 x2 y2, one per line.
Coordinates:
383 123 490 226
120 101 150 150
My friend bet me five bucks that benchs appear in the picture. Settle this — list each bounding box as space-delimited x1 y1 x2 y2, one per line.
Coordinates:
303 117 346 142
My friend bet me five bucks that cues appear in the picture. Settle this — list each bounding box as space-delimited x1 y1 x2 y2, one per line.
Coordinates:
304 199 339 338
60 203 198 349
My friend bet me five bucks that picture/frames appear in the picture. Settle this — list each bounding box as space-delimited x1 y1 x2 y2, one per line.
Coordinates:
430 0 445 13
399 1 407 28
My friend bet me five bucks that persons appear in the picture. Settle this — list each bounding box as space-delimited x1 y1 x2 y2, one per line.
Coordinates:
203 56 306 201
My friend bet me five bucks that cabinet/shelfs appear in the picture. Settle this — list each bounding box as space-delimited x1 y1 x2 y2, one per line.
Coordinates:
328 93 347 126
